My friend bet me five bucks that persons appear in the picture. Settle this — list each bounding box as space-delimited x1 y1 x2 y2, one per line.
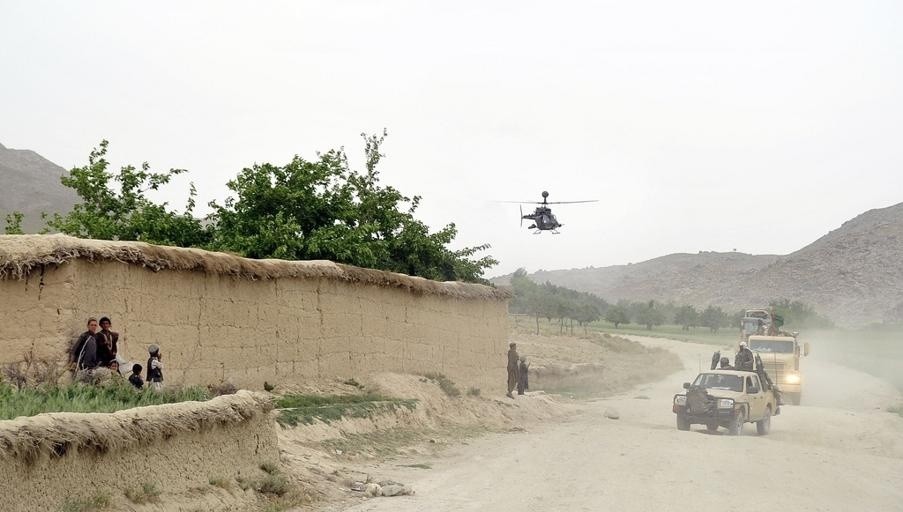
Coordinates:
717 342 757 372
66 317 165 389
506 339 530 399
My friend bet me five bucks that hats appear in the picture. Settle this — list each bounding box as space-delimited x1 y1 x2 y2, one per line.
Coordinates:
148 344 159 354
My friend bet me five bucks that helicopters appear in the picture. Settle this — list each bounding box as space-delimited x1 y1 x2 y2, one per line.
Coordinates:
494 191 598 234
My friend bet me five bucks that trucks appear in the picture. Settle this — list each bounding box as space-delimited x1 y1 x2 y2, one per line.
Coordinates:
739 310 808 409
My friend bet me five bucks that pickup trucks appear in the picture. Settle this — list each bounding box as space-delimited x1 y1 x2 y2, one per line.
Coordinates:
673 366 780 435
